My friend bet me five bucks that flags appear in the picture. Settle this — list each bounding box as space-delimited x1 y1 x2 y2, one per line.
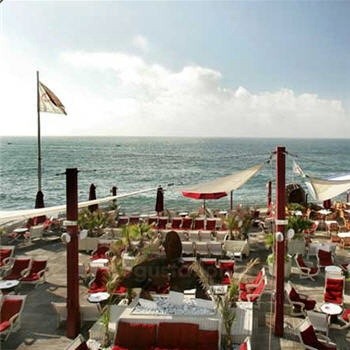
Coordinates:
37 83 68 117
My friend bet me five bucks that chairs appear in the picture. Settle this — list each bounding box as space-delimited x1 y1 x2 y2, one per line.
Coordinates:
0 204 350 347
